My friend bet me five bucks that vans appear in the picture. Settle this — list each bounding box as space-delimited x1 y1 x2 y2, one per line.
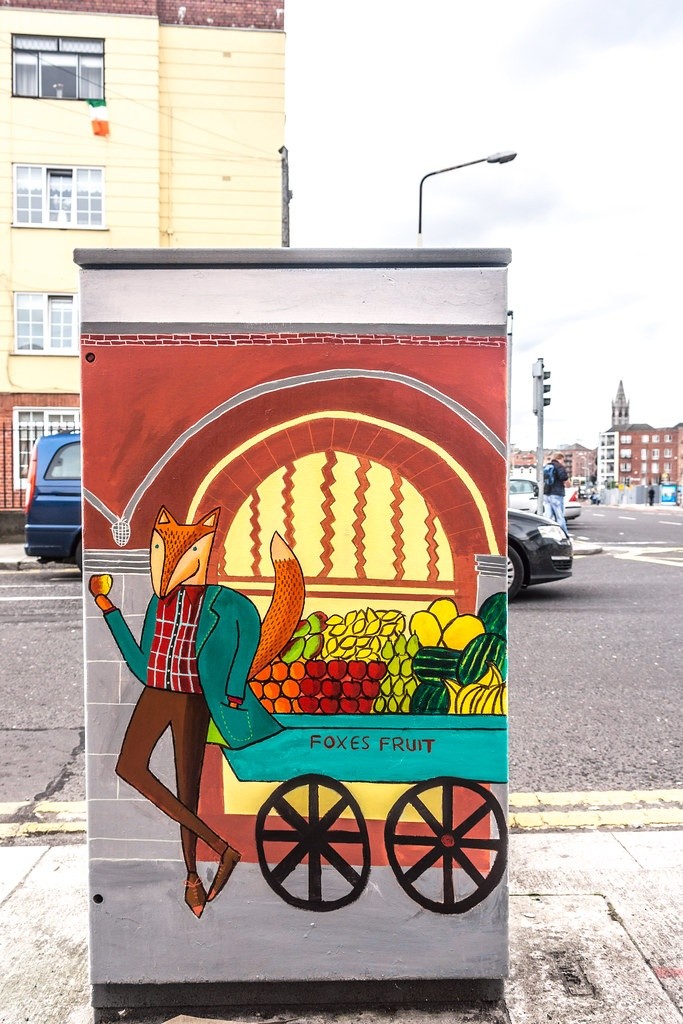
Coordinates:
22 428 81 574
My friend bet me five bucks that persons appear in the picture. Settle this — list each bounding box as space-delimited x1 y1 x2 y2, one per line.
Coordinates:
589 492 598 504
648 487 656 505
543 453 569 535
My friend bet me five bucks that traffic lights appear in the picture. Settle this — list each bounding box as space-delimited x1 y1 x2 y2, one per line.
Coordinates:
533 363 551 415
626 476 631 488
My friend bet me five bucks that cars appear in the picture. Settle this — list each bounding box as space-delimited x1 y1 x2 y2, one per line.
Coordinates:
507 509 574 601
566 487 581 522
509 476 542 516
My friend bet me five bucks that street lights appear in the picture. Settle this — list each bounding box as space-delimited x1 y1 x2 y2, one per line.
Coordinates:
580 456 592 495
419 151 518 241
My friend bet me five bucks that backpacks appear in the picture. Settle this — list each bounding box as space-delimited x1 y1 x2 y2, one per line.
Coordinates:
544 463 558 486
